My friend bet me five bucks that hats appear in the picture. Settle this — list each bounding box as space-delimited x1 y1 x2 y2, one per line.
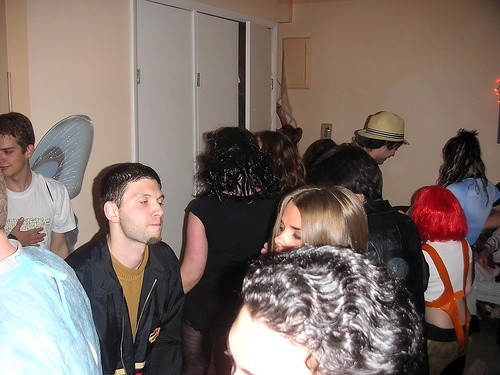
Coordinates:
357 111 408 144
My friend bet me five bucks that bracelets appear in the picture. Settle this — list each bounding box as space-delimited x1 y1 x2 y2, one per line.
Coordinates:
7 234 17 241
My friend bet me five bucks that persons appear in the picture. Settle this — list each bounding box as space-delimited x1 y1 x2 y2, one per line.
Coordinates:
179 111 500 375
0 169 104 375
0 112 77 260
64 162 184 375
